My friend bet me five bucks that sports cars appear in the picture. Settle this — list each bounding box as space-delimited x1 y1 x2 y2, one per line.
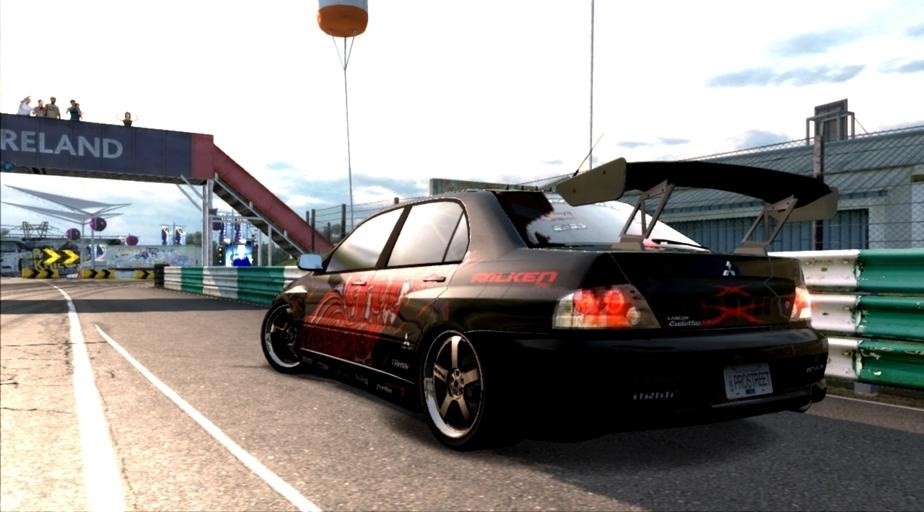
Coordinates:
258 156 842 451
